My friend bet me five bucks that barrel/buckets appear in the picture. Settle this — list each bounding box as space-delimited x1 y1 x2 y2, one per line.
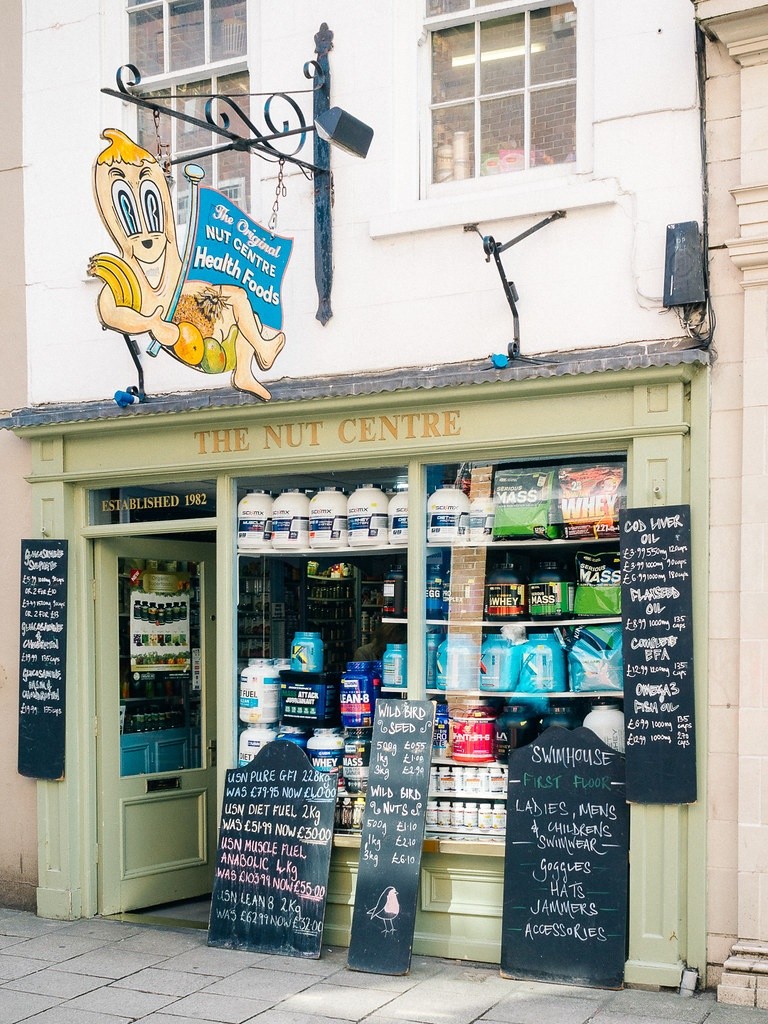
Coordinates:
434 169 454 183
437 145 453 169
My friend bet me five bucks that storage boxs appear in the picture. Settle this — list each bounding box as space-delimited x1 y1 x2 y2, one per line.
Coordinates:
480 153 499 176
498 149 544 174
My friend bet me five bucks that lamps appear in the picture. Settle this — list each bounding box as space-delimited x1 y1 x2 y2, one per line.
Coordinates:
248 106 375 160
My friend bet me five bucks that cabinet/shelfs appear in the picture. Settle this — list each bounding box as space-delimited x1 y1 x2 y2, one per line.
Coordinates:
118 537 623 842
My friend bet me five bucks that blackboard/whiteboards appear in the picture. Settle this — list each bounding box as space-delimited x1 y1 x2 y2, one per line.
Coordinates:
16 538 69 782
498 724 630 990
346 698 437 976
206 739 340 960
617 504 697 806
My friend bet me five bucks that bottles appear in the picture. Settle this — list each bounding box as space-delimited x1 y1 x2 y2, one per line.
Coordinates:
134 600 141 619
165 603 173 623
307 586 350 598
141 601 149 621
157 631 186 644
157 604 165 625
173 602 180 621
127 705 185 732
353 798 366 829
311 623 347 640
334 798 341 829
180 602 187 620
134 631 157 643
324 642 346 663
429 767 509 793
307 603 353 619
426 802 507 830
120 676 181 698
149 602 157 622
341 798 353 829
